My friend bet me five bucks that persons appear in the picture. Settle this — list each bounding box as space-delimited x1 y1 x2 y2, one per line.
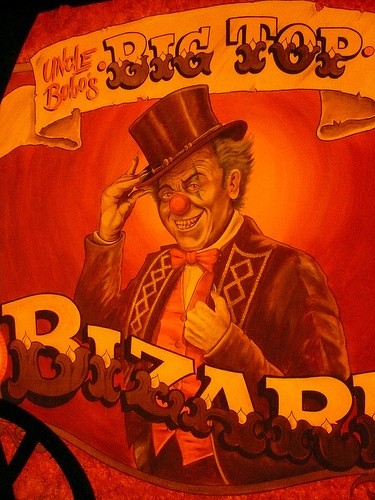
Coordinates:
77 122 353 488
